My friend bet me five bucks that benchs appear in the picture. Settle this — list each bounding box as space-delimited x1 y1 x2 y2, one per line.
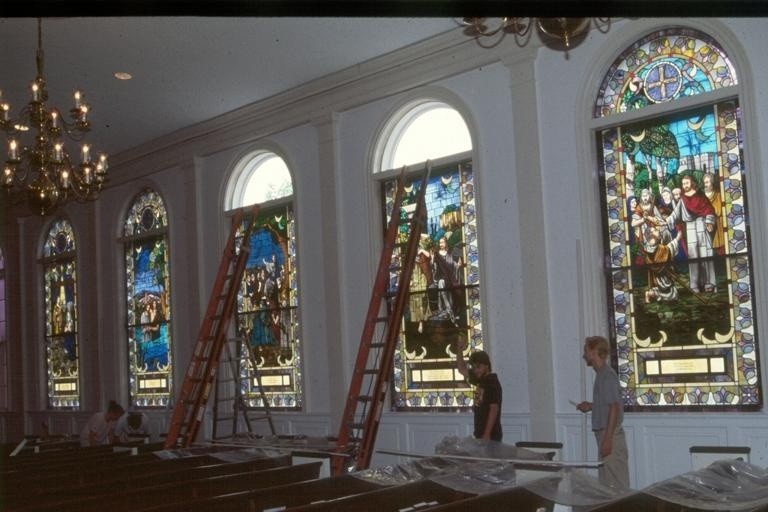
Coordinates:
0 436 768 512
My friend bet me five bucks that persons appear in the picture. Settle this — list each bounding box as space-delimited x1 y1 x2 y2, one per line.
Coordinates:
80 400 125 448
627 173 726 304
408 237 463 333
114 412 152 445
578 336 628 491
457 336 503 442
237 254 285 345
140 299 163 342
53 297 76 360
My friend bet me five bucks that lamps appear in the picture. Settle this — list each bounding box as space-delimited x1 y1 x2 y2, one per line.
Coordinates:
454 16 644 49
1 18 113 217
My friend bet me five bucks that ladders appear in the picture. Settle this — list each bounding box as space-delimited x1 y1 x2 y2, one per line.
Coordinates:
331 160 433 476
164 207 258 449
212 326 276 441
